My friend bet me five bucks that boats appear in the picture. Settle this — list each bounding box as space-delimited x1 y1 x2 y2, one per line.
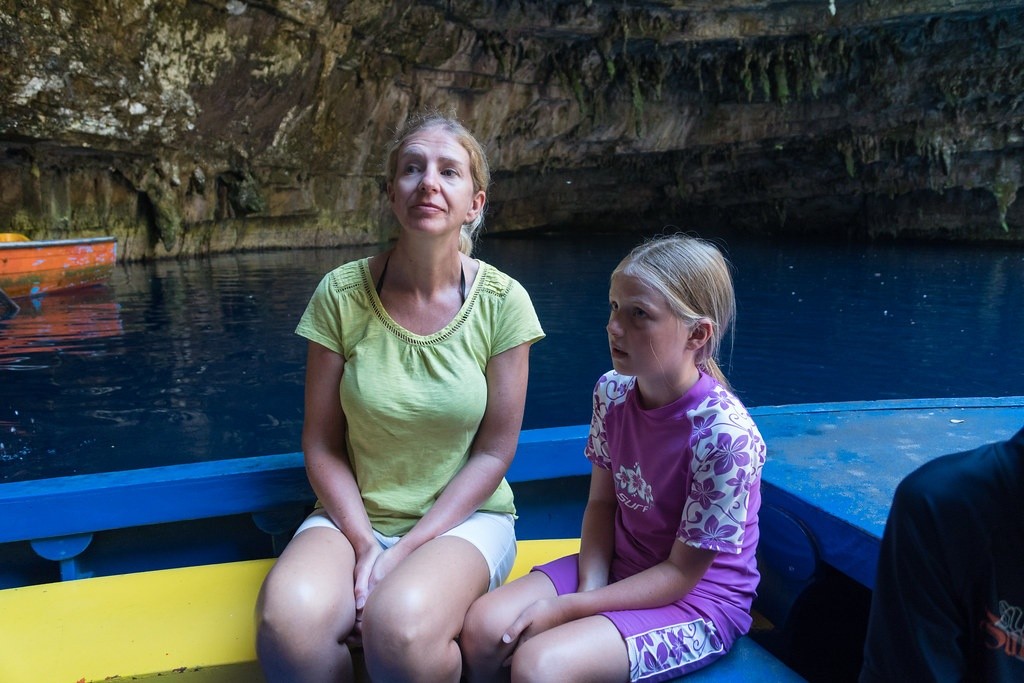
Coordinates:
0 396 1024 683
0 263 116 299
0 236 117 275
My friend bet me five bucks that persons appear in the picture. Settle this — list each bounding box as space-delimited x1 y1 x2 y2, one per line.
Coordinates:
459 237 768 683
253 115 547 682
858 420 1024 683
